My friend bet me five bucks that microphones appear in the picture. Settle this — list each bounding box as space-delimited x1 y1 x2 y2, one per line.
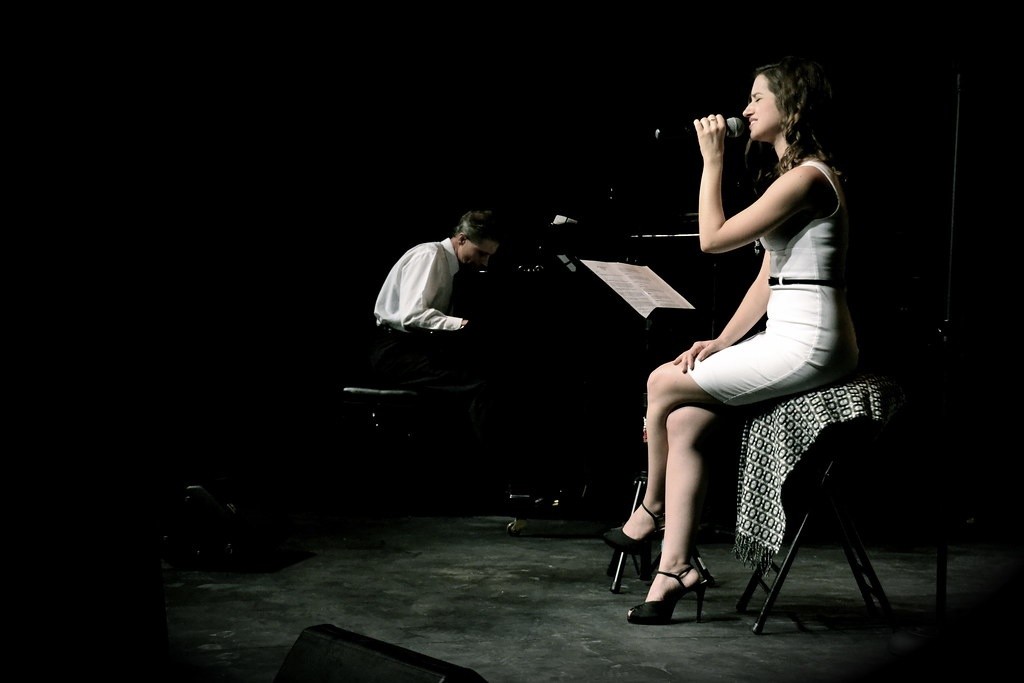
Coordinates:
655 117 745 139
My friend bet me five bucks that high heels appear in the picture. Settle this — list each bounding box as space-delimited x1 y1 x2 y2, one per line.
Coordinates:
627 565 707 625
603 501 665 554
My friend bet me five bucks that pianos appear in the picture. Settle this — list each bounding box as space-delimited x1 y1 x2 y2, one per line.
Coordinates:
425 120 759 535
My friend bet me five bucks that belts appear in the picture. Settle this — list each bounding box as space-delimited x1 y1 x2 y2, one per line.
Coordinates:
769 276 842 288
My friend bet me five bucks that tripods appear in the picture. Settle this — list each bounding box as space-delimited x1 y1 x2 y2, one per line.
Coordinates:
578 258 715 594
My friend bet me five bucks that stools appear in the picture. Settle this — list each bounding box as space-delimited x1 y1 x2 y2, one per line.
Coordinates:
342 387 422 520
736 374 906 635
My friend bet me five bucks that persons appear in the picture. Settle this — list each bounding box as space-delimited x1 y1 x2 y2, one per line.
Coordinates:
602 55 860 625
371 209 560 507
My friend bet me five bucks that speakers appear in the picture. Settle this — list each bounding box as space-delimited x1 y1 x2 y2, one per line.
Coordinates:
271 624 487 683
183 485 269 574
